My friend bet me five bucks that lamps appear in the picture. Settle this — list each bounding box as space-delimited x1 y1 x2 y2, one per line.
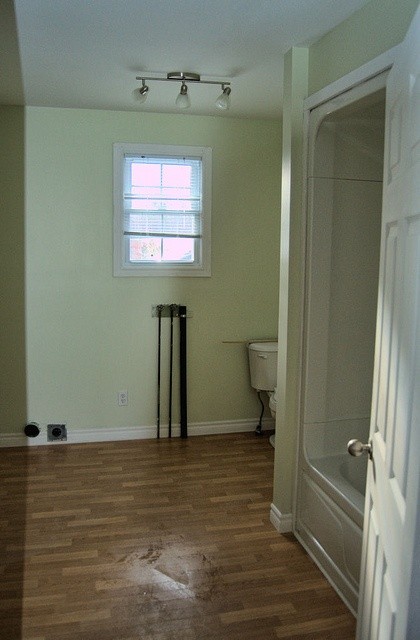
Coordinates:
130 73 231 111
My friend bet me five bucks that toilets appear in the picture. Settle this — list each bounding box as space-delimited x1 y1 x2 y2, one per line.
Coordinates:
248 341 278 450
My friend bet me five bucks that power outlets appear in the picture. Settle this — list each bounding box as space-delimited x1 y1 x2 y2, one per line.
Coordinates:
118 390 128 406
